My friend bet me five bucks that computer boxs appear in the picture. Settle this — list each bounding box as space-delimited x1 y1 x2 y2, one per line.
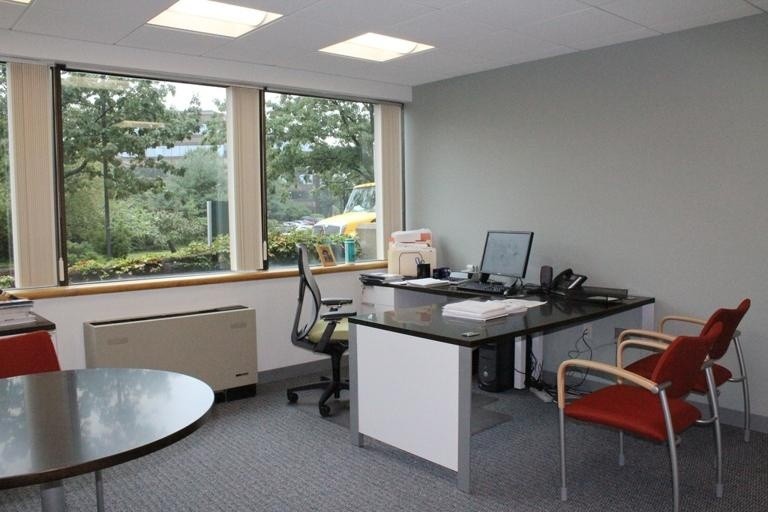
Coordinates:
478 340 514 393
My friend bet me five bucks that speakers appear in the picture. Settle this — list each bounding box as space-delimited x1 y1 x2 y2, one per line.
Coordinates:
540 266 553 291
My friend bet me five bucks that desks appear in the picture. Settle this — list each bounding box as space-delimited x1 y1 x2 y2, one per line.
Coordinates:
0 367 215 512
348 276 655 494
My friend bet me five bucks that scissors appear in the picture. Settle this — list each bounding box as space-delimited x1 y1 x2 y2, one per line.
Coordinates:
415 257 425 264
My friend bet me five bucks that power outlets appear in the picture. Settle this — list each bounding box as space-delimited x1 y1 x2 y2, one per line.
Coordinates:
583 324 592 340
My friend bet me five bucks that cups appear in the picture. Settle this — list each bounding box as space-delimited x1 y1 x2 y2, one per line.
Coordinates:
417 264 430 278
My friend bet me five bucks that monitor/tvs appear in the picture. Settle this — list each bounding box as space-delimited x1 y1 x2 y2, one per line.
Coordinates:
479 231 534 278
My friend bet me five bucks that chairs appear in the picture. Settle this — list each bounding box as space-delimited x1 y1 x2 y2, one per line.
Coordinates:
617 297 752 443
556 320 724 512
286 245 358 418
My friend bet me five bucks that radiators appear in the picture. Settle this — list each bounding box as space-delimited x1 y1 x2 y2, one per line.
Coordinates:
83 304 258 404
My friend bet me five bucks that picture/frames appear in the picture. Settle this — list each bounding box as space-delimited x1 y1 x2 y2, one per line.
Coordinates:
316 245 337 267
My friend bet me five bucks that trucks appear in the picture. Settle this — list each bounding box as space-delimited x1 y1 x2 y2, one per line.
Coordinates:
312 182 375 238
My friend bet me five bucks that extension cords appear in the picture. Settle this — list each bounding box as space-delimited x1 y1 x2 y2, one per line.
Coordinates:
530 387 553 402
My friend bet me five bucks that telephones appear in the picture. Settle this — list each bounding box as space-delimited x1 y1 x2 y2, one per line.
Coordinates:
553 268 589 290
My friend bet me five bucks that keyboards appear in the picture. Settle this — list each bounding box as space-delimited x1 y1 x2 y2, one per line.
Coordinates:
457 281 504 294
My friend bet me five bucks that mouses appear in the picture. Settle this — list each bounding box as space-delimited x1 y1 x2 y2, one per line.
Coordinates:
503 288 518 296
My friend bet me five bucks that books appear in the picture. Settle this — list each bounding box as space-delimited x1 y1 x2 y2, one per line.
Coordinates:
0 297 36 326
440 296 548 325
359 270 450 292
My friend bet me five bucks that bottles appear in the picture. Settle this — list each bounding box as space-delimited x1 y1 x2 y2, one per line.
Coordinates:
344 239 355 263
